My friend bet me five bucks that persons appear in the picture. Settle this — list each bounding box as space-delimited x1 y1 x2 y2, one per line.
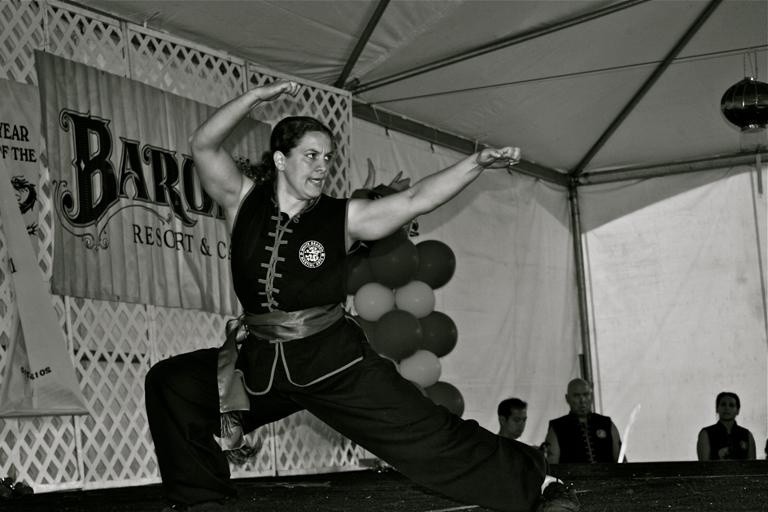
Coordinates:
764 438 768 459
347 184 464 417
544 378 627 463
144 79 582 510
697 391 756 461
497 397 527 439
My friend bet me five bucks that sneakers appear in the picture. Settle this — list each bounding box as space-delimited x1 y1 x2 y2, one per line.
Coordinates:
538 481 582 512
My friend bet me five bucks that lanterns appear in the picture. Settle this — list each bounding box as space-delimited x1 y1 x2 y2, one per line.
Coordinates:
718 75 768 135
